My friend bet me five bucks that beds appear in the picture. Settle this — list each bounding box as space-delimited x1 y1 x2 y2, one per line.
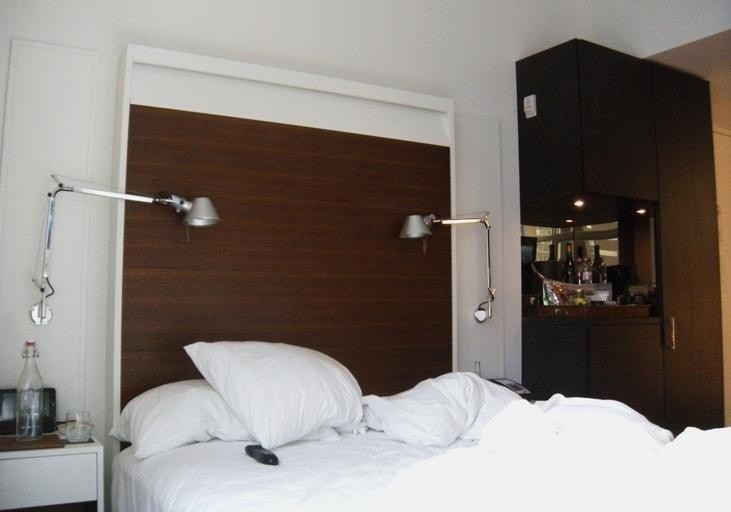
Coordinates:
110 369 731 510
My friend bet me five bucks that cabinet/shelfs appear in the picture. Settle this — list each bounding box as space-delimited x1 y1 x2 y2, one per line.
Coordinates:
516 39 725 430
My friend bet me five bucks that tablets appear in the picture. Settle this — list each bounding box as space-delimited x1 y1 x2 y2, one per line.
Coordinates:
0 387 56 436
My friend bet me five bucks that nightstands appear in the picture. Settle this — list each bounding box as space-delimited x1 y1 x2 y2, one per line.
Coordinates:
0 420 105 511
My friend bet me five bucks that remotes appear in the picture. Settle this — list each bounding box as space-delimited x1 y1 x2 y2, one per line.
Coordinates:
246 445 278 465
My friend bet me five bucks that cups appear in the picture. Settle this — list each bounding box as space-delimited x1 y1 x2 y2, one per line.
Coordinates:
65 410 91 444
616 288 658 305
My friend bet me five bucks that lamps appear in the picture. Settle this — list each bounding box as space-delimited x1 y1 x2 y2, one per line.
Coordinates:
30 170 221 324
398 209 494 324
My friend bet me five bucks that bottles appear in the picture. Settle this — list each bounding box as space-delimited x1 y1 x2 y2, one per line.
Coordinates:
15 341 44 443
562 242 607 283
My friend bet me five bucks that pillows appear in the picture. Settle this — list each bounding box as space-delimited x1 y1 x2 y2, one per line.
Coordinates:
182 340 365 452
111 380 257 461
